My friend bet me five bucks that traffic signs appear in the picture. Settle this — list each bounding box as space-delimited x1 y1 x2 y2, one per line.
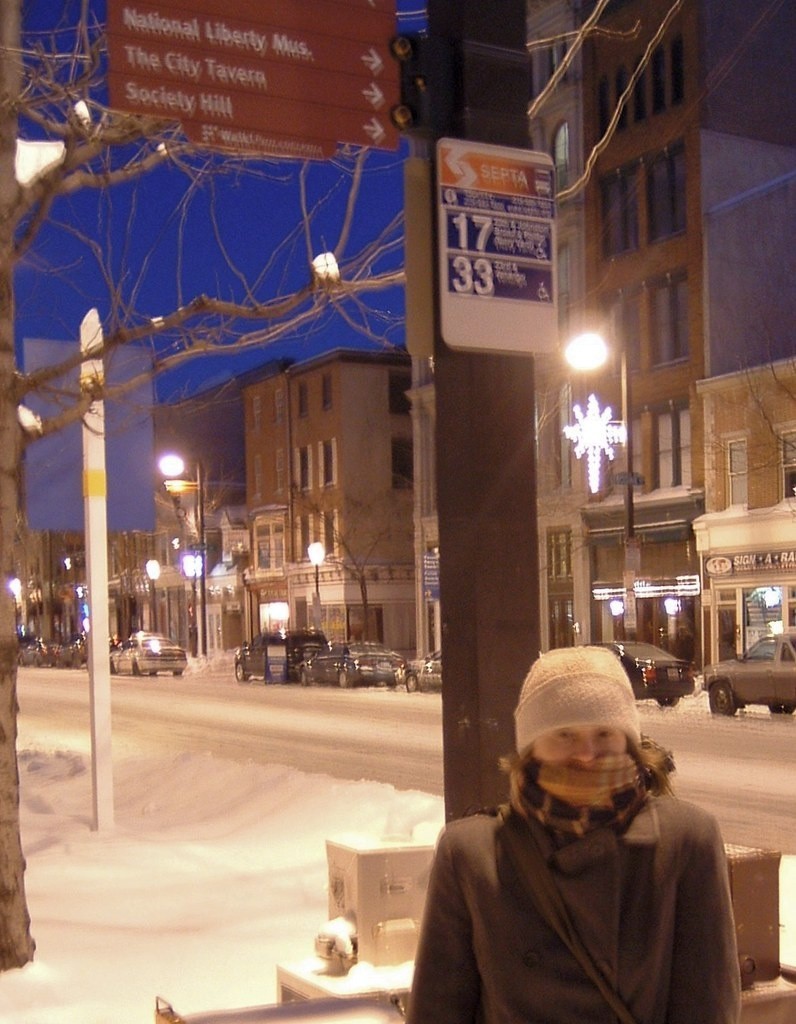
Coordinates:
108 0 401 83
108 72 400 153
105 33 403 115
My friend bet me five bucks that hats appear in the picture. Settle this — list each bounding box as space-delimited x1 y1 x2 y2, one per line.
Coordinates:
513 646 642 760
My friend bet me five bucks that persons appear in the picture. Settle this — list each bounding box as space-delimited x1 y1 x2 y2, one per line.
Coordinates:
676 627 696 664
405 646 741 1024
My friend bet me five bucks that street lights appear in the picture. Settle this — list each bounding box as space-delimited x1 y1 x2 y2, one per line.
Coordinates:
563 328 635 639
157 454 208 658
307 542 325 596
145 558 160 633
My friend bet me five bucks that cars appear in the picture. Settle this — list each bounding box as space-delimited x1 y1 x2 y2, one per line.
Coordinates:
584 640 697 708
109 631 187 677
402 650 442 693
298 639 408 690
17 631 89 669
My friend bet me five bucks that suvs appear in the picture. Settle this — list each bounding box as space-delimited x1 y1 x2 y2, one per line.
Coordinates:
234 629 329 684
702 633 796 715
